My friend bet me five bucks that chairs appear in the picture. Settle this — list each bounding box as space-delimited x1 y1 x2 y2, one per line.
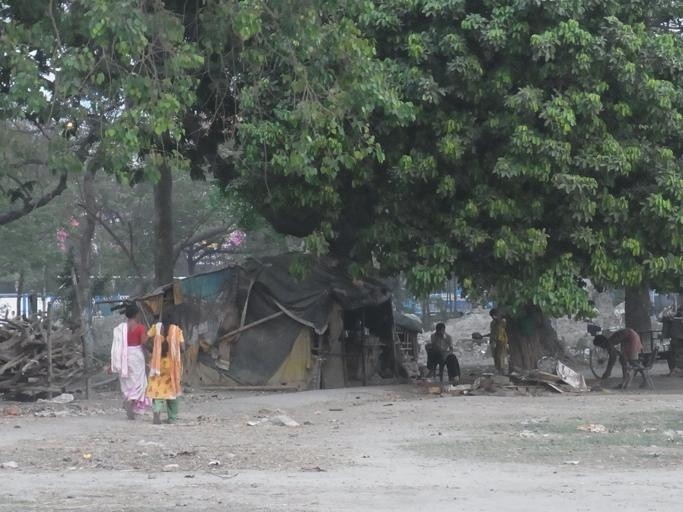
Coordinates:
621 346 658 393
424 343 450 382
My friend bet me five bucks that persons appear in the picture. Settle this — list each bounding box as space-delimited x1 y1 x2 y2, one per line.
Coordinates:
593 328 642 389
431 323 461 384
111 305 151 419
143 305 185 424
481 308 508 375
